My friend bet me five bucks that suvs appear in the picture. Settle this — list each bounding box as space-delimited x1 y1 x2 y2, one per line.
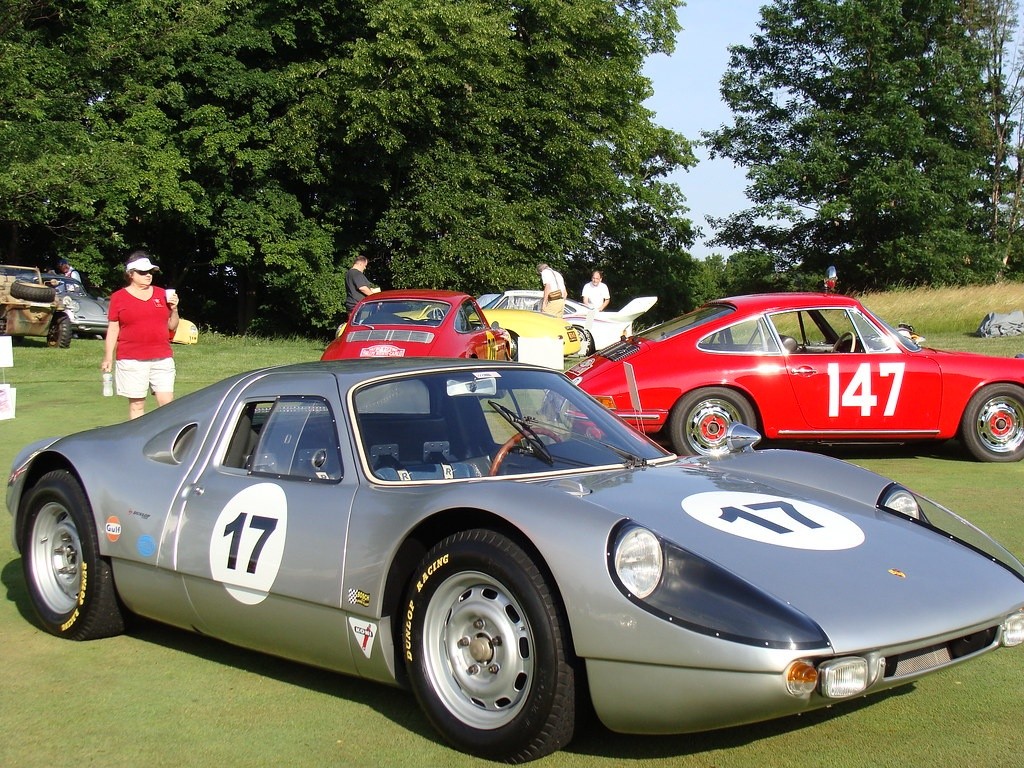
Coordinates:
0 265 72 352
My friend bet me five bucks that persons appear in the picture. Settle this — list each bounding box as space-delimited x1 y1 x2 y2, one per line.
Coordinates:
102 251 179 419
537 264 568 317
345 256 373 316
582 271 610 311
59 260 80 282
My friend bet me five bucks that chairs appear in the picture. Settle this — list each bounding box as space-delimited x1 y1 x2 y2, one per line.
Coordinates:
768 335 799 353
361 311 404 324
245 418 446 471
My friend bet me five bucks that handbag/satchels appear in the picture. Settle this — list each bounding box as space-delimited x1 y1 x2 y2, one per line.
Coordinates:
548 290 562 301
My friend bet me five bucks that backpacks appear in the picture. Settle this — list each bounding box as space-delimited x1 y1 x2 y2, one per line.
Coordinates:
69 270 91 293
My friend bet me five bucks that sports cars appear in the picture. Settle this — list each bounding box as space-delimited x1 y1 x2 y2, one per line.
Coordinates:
324 287 514 403
4 356 1023 768
479 291 659 355
170 319 199 346
16 273 111 339
544 291 1023 466
335 306 580 361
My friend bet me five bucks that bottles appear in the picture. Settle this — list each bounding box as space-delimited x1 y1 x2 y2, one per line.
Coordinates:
103 371 113 397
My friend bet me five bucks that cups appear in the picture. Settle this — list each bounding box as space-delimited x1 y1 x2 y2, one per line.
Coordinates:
165 289 176 303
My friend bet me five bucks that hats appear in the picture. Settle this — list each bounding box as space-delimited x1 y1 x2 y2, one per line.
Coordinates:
57 259 67 264
126 258 160 272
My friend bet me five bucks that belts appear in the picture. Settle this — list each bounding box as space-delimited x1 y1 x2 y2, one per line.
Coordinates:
558 297 564 299
